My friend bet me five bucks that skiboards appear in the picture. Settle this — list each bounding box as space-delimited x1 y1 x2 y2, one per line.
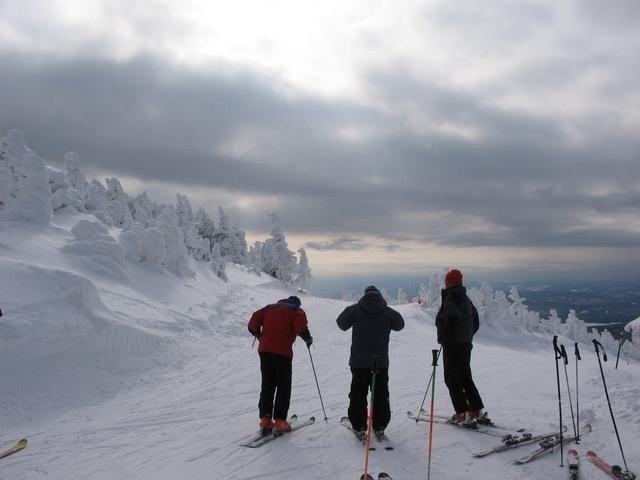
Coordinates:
340 414 395 451
407 409 524 438
476 424 592 464
239 414 315 447
567 449 635 480
1 439 26 458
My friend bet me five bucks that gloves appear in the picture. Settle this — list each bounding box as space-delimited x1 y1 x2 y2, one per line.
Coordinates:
308 338 313 346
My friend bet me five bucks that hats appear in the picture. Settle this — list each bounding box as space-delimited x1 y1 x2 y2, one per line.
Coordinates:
288 296 302 306
364 286 377 293
446 270 462 284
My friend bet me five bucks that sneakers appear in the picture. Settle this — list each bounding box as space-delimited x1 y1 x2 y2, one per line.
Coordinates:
275 419 290 431
261 417 274 427
468 409 480 418
372 421 384 436
351 424 364 437
449 413 465 422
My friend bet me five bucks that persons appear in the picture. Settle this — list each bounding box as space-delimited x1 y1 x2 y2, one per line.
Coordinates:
248 295 313 437
434 270 484 423
337 285 405 432
